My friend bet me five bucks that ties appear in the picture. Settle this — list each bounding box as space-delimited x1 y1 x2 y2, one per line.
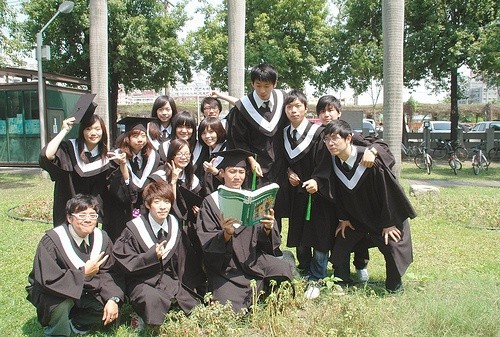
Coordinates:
80 240 87 254
292 129 298 144
162 130 167 138
157 228 167 244
343 162 349 172
263 101 270 112
133 156 139 170
209 153 215 163
85 152 92 163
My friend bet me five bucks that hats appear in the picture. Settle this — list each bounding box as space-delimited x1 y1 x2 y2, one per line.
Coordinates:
211 148 259 191
114 116 157 134
64 93 98 125
287 180 322 221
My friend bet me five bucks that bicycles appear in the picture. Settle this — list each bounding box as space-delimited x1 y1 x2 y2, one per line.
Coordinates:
434 138 468 163
413 140 433 176
468 142 489 176
441 139 462 176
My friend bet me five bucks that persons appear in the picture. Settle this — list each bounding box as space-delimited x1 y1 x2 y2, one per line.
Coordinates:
194 88 243 141
194 148 297 316
39 113 111 229
151 95 177 162
191 116 231 199
304 93 397 302
274 92 334 276
25 194 126 337
110 181 204 337
105 62 289 247
322 119 417 296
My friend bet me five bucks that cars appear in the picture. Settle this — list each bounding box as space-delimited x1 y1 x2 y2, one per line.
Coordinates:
352 118 384 136
417 120 467 141
470 121 500 144
458 122 473 132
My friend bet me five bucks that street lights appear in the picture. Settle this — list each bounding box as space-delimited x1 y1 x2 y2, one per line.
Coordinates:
35 0 74 152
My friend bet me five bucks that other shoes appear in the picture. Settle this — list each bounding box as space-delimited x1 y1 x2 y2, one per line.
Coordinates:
332 283 346 296
355 267 369 282
296 268 310 282
304 284 321 299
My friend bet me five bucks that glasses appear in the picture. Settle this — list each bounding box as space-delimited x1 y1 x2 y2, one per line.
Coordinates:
204 106 218 110
176 153 191 158
71 213 98 220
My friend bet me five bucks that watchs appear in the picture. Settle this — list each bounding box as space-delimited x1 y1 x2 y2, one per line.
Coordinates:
368 146 379 158
109 295 122 308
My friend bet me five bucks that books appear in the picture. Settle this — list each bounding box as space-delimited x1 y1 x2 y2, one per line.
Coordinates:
216 183 280 227
